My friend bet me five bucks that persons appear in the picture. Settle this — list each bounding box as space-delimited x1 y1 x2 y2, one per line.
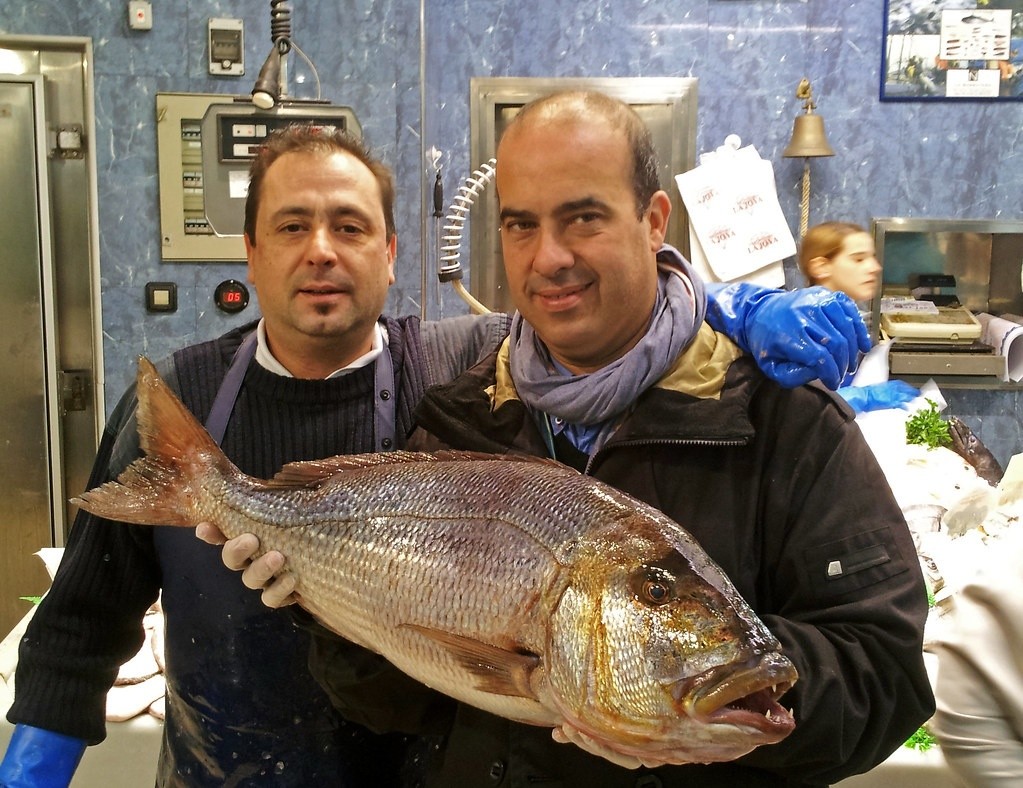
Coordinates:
933 524 1023 788
0 128 870 788
194 89 936 788
799 220 921 415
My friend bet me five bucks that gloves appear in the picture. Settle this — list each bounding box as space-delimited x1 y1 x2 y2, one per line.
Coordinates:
704 281 872 393
0 723 86 788
835 379 921 415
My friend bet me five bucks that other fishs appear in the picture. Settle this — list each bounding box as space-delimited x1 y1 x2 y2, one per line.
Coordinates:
63 347 799 763
